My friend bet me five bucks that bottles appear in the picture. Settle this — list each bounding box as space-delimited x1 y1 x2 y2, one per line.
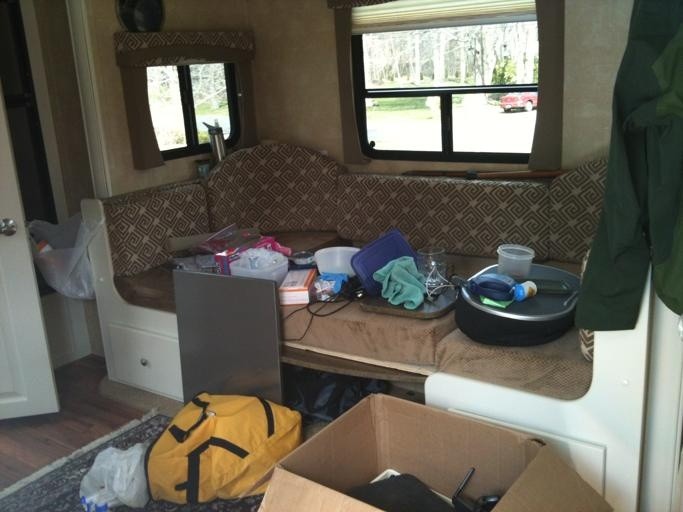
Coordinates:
202 120 228 161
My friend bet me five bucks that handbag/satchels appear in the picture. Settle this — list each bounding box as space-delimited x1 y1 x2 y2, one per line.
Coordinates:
142 387 305 503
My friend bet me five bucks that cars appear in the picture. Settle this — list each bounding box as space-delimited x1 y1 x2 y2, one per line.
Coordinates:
497 91 538 113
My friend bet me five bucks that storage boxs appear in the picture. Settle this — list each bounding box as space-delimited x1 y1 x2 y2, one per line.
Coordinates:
257 393 613 512
229 258 288 289
278 267 317 305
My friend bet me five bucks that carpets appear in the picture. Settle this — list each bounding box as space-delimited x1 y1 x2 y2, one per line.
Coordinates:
0 407 264 510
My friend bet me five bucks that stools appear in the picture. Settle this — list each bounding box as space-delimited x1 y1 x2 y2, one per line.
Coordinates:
279 299 460 383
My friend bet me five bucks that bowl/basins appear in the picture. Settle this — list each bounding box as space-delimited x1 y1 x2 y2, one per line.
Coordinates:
230 256 289 285
312 246 361 277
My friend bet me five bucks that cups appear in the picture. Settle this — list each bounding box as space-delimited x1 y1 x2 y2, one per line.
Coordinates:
417 247 448 298
196 160 213 177
497 242 535 280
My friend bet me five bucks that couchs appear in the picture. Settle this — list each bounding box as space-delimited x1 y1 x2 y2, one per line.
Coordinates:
80 139 654 512
